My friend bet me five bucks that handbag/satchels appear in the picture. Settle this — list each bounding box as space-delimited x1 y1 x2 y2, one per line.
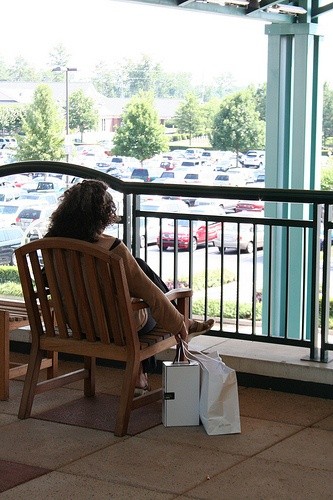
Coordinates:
162 338 241 434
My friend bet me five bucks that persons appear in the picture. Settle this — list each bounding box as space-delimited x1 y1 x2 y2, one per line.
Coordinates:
41 180 214 397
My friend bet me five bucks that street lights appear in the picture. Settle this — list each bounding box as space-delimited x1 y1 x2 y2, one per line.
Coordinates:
51 66 78 163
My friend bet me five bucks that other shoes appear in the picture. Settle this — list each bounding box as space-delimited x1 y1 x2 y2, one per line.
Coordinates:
185 319 214 342
135 383 152 395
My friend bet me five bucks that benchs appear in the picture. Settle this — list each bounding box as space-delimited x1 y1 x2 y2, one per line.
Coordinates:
1 300 58 401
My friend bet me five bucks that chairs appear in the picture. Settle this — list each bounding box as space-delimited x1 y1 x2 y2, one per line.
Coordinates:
14 238 193 437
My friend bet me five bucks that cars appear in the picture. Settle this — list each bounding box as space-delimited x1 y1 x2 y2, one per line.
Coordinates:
322 148 333 157
0 226 27 266
319 204 333 251
0 137 265 268
156 220 222 252
214 211 264 254
